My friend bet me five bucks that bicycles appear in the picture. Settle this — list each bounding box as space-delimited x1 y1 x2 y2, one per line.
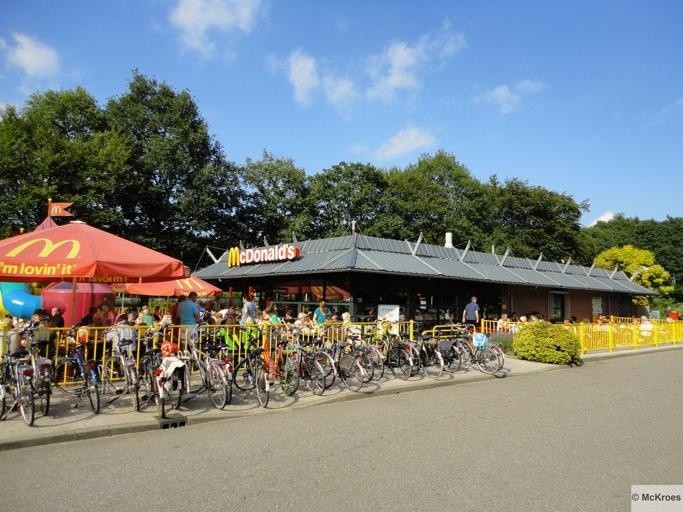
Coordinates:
21 317 56 418
101 319 140 413
53 321 101 414
0 330 36 427
0 360 9 420
140 315 508 429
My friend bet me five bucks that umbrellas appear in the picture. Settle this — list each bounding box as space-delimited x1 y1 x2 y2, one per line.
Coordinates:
109 272 221 296
278 286 350 300
0 220 191 326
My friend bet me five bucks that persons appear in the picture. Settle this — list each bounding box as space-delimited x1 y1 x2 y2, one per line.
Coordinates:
639 316 653 344
496 313 544 334
6 292 378 379
595 314 603 324
399 307 454 320
462 296 480 331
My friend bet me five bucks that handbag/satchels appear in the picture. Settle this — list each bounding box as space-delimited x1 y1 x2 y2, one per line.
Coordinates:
156 356 191 398
439 340 458 360
473 333 488 349
